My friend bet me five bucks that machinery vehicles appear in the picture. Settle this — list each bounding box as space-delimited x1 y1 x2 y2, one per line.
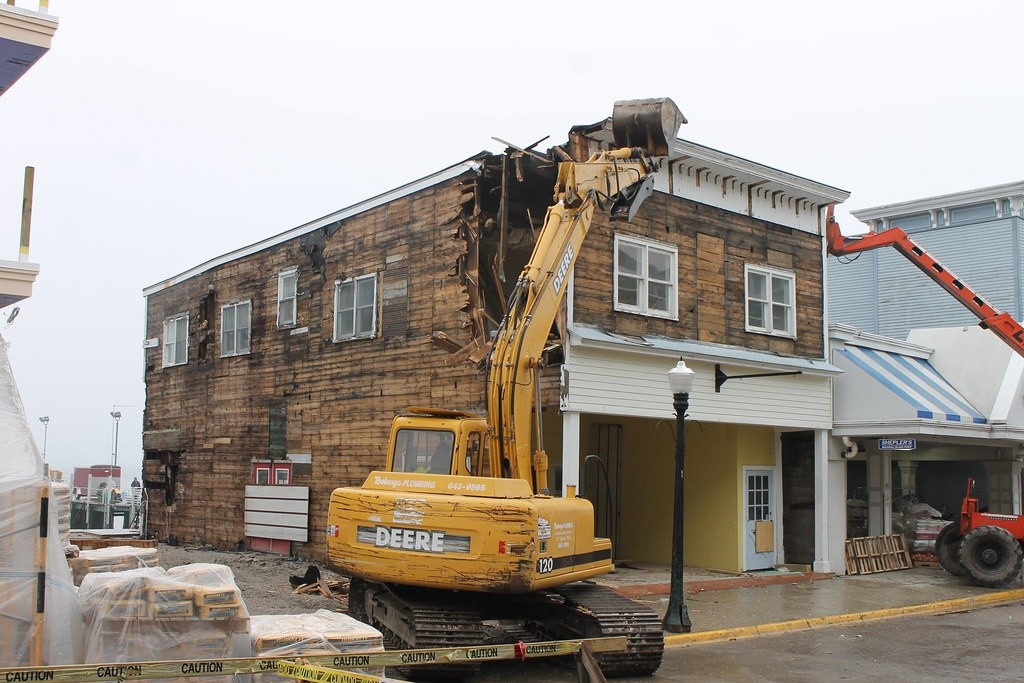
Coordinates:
316 95 694 676
825 200 1024 589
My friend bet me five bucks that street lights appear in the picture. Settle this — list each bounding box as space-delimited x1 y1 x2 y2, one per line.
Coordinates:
108 410 122 467
39 416 51 461
659 355 698 633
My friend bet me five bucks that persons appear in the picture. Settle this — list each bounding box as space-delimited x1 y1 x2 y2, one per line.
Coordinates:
131 475 141 503
428 441 450 474
